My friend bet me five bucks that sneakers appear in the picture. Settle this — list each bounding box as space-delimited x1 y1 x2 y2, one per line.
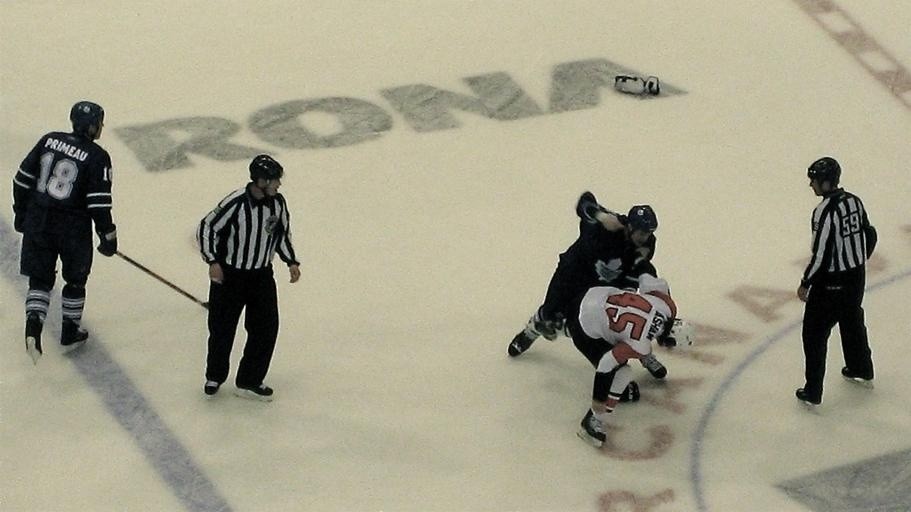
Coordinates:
796 389 822 404
508 312 543 357
842 366 874 379
640 356 667 379
236 379 273 397
206 379 220 395
580 410 607 443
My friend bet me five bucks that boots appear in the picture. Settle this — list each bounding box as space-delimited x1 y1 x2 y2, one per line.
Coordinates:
25 313 42 356
61 324 88 345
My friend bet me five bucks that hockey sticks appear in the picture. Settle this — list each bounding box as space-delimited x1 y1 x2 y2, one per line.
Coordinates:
113 250 211 311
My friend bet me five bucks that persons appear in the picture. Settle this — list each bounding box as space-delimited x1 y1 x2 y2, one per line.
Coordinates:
11 101 117 354
195 154 301 399
796 157 878 405
507 191 669 377
566 272 694 444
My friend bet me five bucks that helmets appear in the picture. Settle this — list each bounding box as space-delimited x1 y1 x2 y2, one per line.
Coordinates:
667 318 695 348
249 154 282 182
71 101 103 135
629 204 658 233
807 157 841 185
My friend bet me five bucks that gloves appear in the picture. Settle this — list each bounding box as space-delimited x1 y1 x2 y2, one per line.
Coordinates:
96 225 117 256
13 205 25 234
